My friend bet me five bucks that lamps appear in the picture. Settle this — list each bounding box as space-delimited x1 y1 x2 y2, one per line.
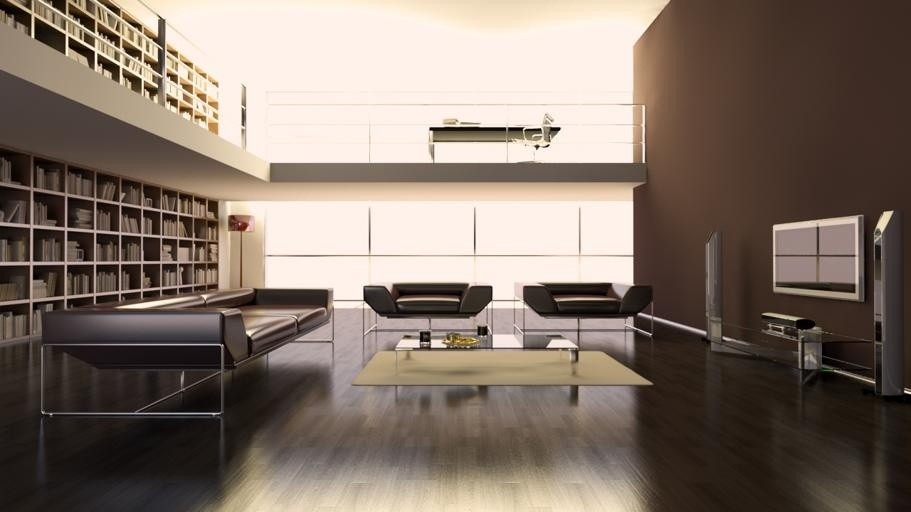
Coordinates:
226 213 258 287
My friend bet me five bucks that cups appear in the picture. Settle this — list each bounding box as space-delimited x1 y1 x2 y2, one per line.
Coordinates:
476 323 487 342
419 329 431 346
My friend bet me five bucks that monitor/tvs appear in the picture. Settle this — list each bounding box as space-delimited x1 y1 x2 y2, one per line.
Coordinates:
772 215 865 302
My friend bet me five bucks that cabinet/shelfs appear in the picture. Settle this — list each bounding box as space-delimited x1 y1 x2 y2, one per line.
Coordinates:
0 149 219 345
0 2 219 139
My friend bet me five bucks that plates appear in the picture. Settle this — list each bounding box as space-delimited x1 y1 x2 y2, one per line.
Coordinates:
443 336 479 349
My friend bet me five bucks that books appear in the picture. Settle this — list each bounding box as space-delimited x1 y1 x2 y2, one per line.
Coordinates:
0 157 219 339
0 0 217 131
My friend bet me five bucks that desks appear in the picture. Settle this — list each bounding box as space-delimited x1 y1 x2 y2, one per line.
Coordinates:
429 124 561 146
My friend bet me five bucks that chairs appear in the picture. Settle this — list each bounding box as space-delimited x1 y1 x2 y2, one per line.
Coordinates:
514 114 555 163
362 282 492 359
513 280 655 348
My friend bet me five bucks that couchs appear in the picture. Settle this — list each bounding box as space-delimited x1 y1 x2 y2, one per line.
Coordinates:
39 287 336 460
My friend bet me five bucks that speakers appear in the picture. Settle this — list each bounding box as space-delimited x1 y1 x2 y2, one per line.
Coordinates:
704 230 723 341
873 210 905 396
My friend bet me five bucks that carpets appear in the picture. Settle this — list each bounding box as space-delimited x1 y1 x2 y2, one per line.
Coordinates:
349 349 655 387
